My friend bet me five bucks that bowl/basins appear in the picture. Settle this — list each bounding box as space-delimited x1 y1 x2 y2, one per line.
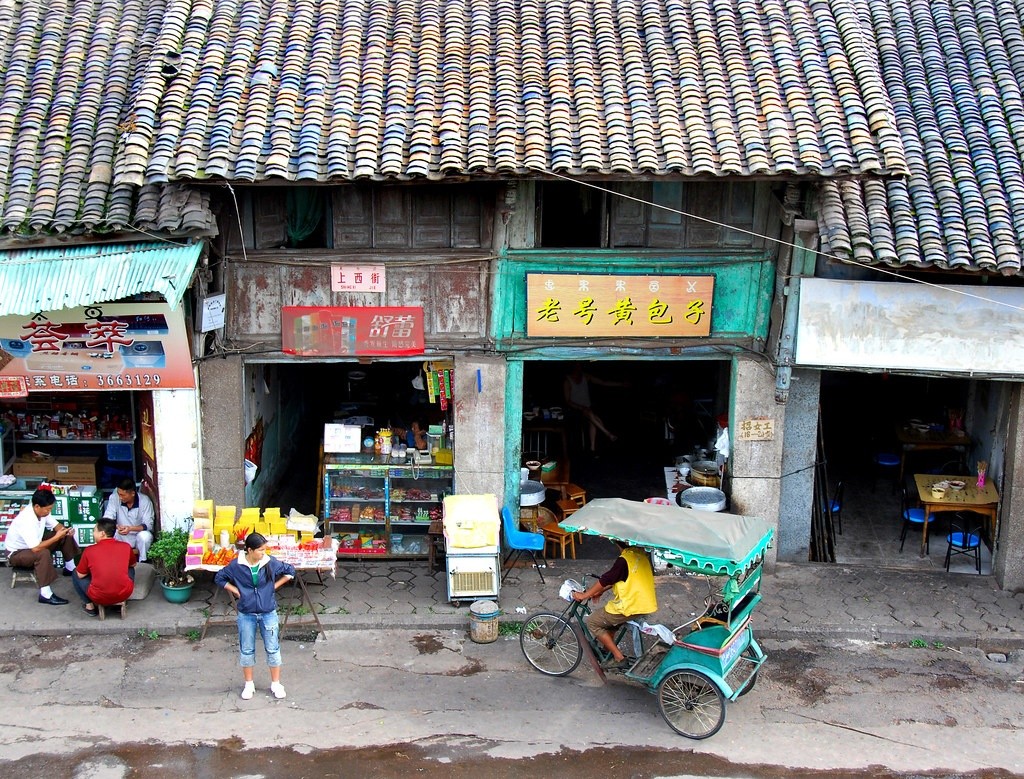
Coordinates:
909 419 922 429
949 481 966 491
931 487 946 499
526 461 541 470
916 424 931 433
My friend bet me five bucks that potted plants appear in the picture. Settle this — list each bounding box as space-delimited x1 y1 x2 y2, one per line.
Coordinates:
145 526 196 604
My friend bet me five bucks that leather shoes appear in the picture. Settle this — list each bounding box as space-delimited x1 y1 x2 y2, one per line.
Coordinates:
39 592 68 605
63 567 73 576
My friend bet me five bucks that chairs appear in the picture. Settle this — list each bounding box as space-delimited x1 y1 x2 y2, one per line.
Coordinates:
943 509 984 575
899 481 935 556
500 506 548 584
823 481 843 545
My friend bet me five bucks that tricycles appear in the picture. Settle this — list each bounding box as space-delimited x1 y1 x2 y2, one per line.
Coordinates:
519 496 774 740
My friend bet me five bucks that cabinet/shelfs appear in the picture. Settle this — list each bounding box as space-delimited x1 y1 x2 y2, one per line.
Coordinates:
0 475 48 567
0 391 137 492
322 453 453 562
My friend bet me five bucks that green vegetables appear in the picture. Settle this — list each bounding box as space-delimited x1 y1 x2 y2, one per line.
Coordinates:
498 622 536 635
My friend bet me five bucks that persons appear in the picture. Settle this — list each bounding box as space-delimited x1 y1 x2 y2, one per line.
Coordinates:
73 518 137 616
405 418 427 450
572 540 658 669
215 532 295 699
4 489 82 605
103 476 154 562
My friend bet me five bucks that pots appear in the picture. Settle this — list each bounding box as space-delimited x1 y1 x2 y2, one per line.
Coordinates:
690 460 719 489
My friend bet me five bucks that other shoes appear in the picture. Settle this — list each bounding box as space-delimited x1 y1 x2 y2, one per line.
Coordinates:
81 604 97 617
600 658 629 669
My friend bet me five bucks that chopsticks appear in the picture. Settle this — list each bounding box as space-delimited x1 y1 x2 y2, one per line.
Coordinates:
949 409 965 419
978 461 987 470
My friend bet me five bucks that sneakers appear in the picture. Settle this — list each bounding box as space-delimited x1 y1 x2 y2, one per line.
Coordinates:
241 683 255 700
270 683 286 698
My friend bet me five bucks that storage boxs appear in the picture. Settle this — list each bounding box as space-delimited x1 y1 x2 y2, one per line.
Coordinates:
541 461 556 472
70 522 99 547
436 448 453 465
324 423 362 453
13 457 55 482
67 489 104 524
54 456 101 485
51 494 68 520
185 499 313 565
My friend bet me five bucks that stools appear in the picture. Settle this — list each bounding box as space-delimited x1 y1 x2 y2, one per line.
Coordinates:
10 569 40 589
542 522 576 560
567 484 587 506
557 499 583 544
98 599 127 621
873 454 901 468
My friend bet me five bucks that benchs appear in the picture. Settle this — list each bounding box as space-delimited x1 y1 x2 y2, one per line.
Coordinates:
681 592 761 651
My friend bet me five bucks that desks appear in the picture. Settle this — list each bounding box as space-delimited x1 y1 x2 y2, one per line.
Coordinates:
664 466 692 506
184 537 339 641
914 474 1000 557
896 420 970 481
530 459 569 499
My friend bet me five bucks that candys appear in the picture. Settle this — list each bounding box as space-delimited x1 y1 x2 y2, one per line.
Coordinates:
380 427 390 432
